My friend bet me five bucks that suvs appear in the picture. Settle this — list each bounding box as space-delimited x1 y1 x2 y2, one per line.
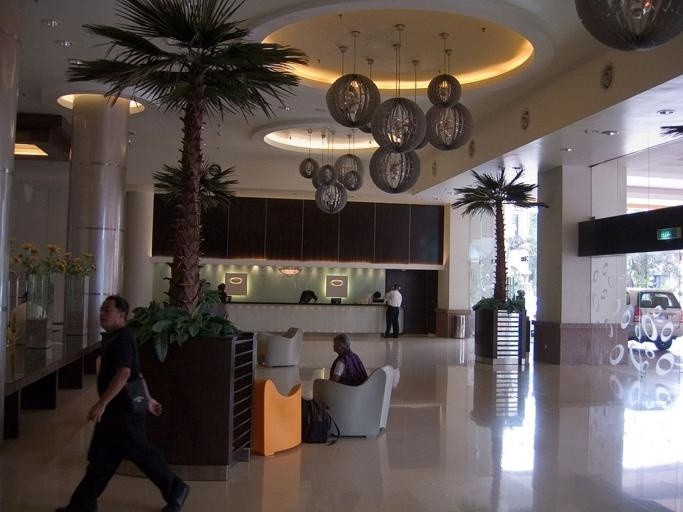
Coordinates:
627 288 683 348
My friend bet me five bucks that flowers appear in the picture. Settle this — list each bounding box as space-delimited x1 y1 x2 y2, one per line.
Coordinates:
12 243 67 316
59 251 97 312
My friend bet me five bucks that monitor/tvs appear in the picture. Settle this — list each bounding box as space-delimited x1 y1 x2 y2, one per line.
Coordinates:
226 296 232 301
373 298 384 303
331 298 341 304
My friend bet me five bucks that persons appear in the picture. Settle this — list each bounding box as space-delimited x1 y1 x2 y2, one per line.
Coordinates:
367 291 381 305
217 283 226 304
8 345 53 380
5 289 47 344
53 295 195 512
329 331 369 386
382 284 403 336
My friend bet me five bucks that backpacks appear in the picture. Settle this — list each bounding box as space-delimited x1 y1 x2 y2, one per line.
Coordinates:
303 399 331 444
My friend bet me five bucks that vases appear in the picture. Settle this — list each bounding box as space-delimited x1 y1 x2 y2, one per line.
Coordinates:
26 271 55 348
63 273 89 336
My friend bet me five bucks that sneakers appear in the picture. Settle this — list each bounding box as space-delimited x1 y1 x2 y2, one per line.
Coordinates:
162 484 189 512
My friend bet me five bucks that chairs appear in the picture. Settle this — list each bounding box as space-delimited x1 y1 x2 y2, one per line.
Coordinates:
257 327 303 368
312 363 395 439
251 377 302 457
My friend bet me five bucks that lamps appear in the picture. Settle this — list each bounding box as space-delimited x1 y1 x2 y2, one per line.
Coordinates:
575 0 683 51
298 22 474 215
